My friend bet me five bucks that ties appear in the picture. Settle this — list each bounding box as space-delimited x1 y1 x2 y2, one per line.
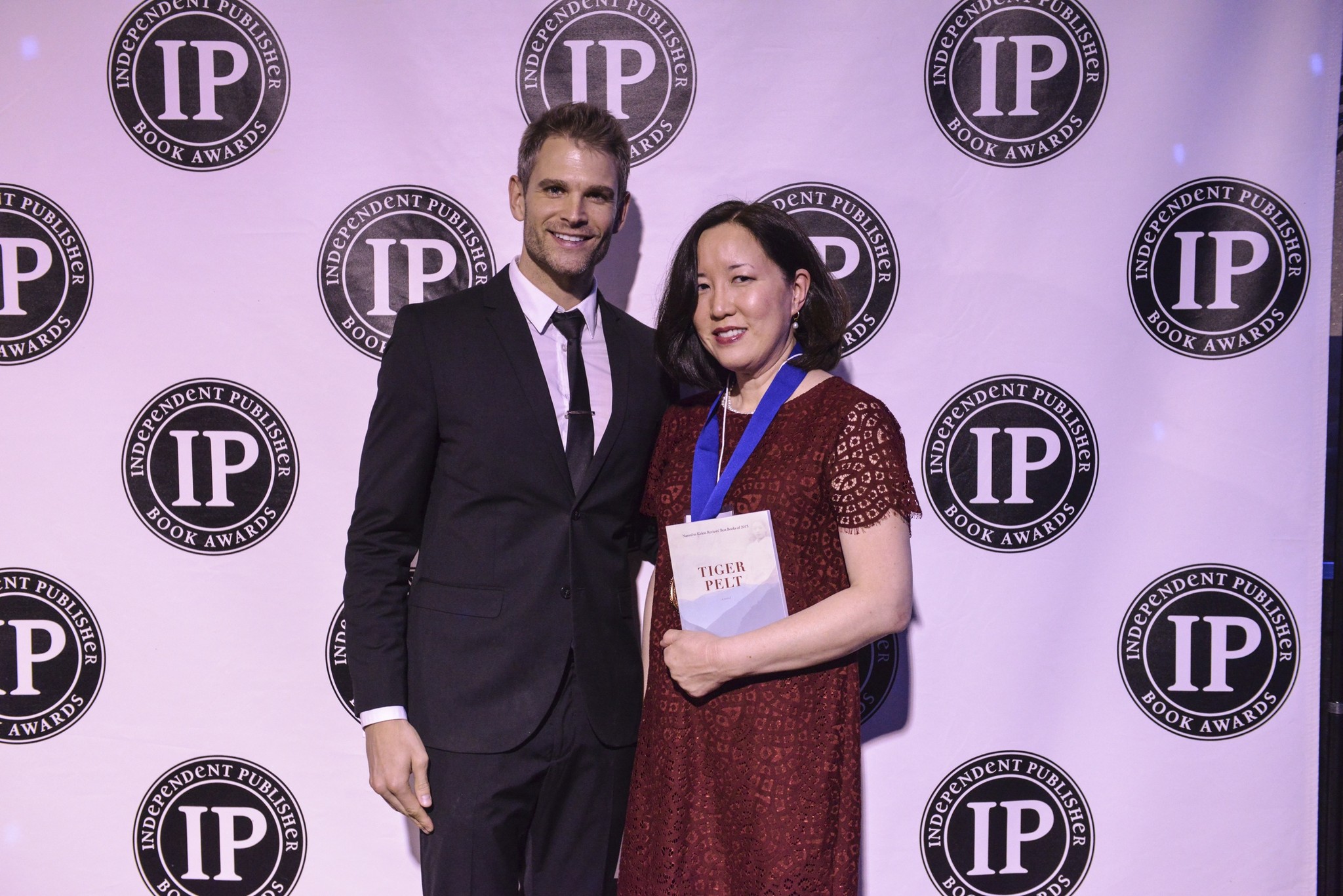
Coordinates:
549 311 594 501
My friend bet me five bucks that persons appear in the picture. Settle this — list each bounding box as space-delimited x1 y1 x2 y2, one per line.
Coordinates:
345 102 671 896
616 200 923 896
